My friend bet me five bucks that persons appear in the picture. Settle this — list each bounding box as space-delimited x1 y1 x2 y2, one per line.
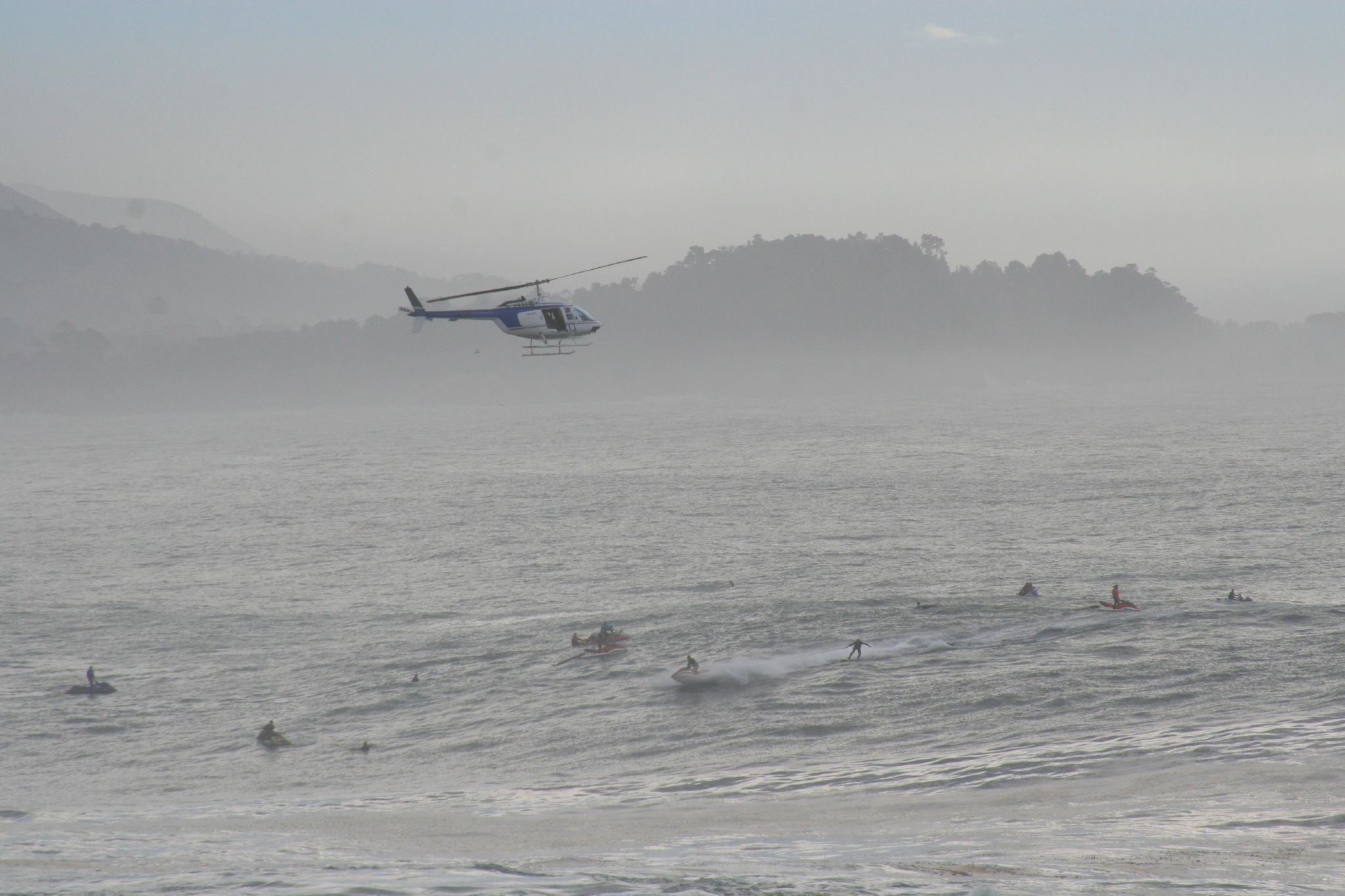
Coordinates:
570 621 612 651
1022 583 1037 596
412 674 420 682
1112 584 1120 609
686 655 699 674
264 721 276 740
845 639 872 660
87 667 95 686
1228 589 1240 600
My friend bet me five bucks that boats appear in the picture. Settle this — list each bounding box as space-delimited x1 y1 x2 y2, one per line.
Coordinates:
66 682 118 695
257 725 302 750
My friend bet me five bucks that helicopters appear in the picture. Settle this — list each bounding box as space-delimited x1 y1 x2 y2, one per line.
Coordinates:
391 254 649 360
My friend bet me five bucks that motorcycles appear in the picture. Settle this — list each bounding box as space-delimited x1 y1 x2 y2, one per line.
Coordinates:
1225 593 1259 603
569 626 635 658
673 667 716 686
1013 591 1042 597
1098 598 1143 612
